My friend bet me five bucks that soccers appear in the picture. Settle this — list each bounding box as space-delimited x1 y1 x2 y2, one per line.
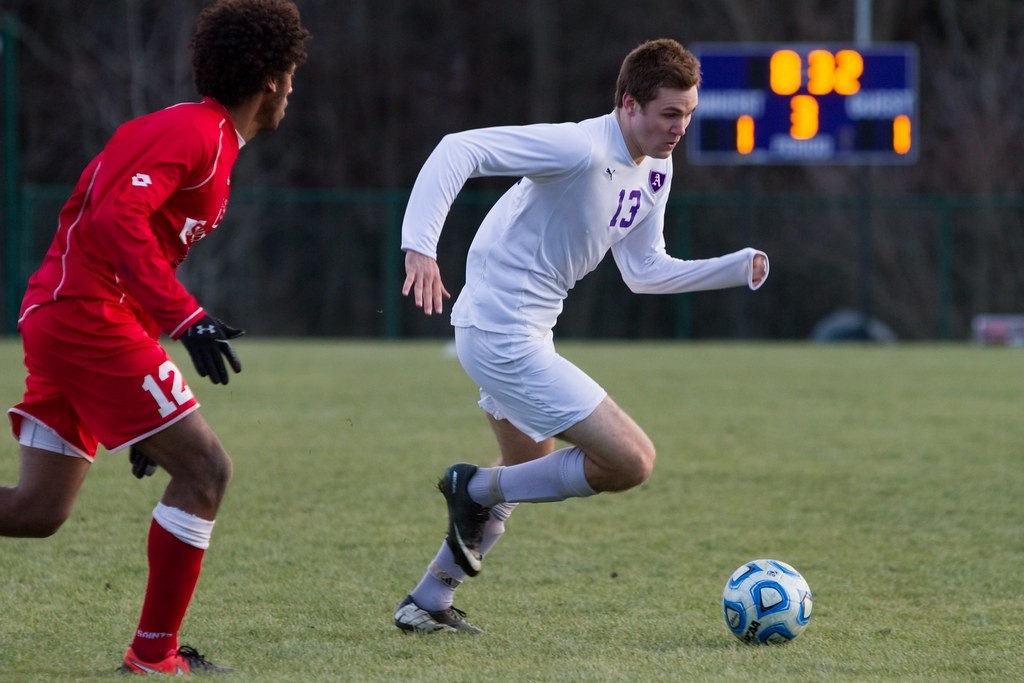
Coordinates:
722 559 812 645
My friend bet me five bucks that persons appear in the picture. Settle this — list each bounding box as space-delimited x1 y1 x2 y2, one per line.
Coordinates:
0 1 311 677
380 37 778 639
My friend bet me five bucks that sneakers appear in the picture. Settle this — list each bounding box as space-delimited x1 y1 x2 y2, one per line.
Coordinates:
436 461 492 578
394 595 492 643
116 643 235 677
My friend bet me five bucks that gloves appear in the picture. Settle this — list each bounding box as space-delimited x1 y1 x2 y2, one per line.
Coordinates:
126 441 163 484
173 309 244 388
